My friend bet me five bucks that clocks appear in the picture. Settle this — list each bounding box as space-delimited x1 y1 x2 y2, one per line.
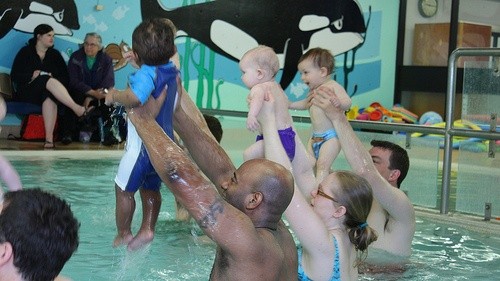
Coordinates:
417 0 439 19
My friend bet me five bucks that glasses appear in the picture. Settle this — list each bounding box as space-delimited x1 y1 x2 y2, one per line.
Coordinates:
317 189 339 203
84 42 98 48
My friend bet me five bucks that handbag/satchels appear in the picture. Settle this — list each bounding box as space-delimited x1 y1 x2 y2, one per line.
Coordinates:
96 104 128 147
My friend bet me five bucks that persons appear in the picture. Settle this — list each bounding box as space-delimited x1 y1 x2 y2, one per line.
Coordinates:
246 87 378 281
10 24 95 148
125 73 298 281
61 32 115 146
239 45 296 162
0 187 81 281
289 47 351 185
307 86 415 273
104 17 224 251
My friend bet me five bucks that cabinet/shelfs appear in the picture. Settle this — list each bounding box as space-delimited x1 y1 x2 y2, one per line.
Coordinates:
408 22 491 122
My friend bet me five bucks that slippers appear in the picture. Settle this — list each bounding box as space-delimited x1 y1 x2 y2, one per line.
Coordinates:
77 105 94 123
43 141 55 148
